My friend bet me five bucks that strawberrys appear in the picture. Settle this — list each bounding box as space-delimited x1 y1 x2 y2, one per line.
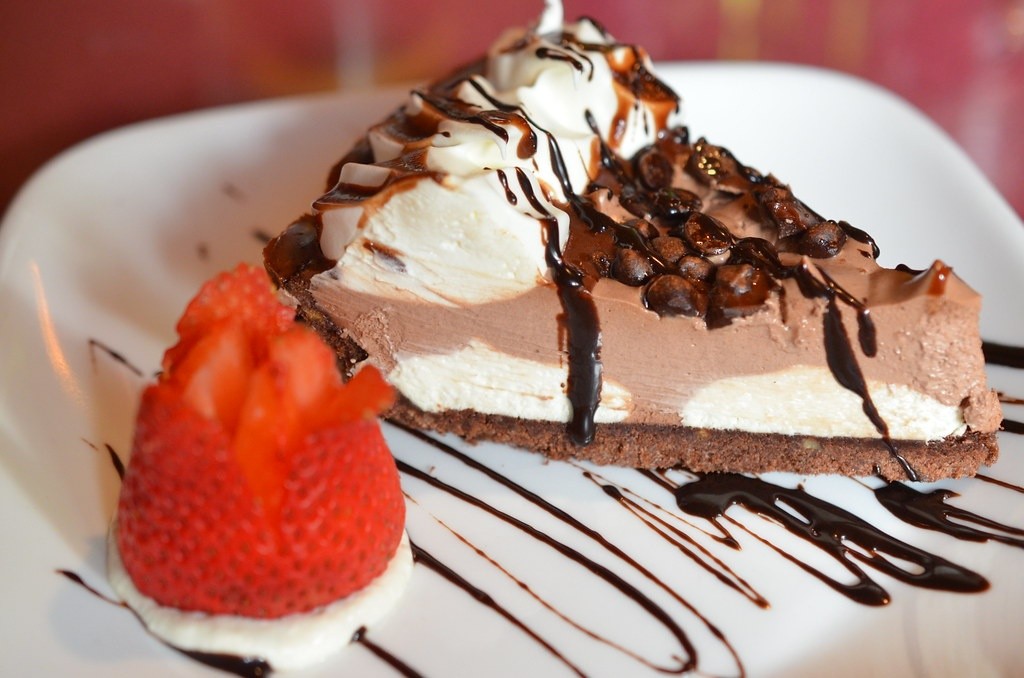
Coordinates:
113 263 405 617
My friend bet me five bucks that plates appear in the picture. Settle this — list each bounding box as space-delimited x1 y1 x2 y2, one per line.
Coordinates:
0 60 1024 678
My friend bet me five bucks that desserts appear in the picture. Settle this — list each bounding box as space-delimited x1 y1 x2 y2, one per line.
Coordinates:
261 0 1007 484
